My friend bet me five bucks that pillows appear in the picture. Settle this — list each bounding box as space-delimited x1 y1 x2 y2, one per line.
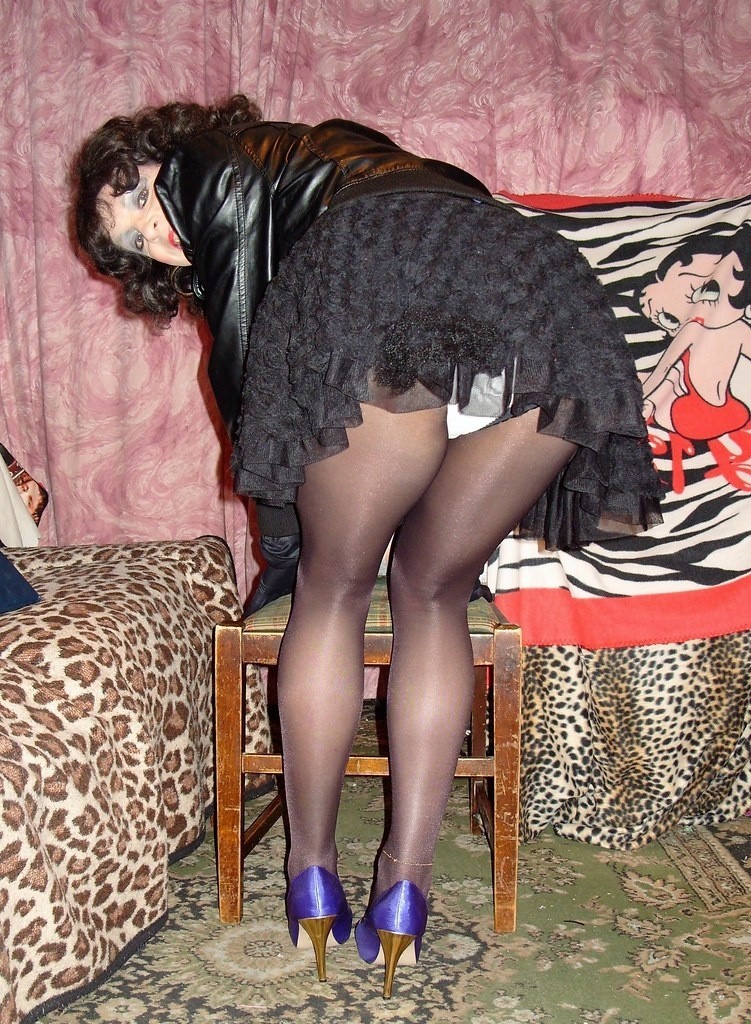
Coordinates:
1 551 44 615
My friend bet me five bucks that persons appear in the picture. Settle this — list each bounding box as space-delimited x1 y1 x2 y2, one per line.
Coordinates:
69 91 668 1001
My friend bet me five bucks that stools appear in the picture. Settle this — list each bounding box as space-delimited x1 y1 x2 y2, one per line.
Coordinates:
212 577 523 937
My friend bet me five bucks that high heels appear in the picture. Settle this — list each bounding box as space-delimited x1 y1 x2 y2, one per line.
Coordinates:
355 879 427 999
284 867 354 983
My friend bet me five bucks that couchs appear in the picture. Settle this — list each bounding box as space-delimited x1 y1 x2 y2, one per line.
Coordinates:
464 190 751 850
0 536 282 1023
